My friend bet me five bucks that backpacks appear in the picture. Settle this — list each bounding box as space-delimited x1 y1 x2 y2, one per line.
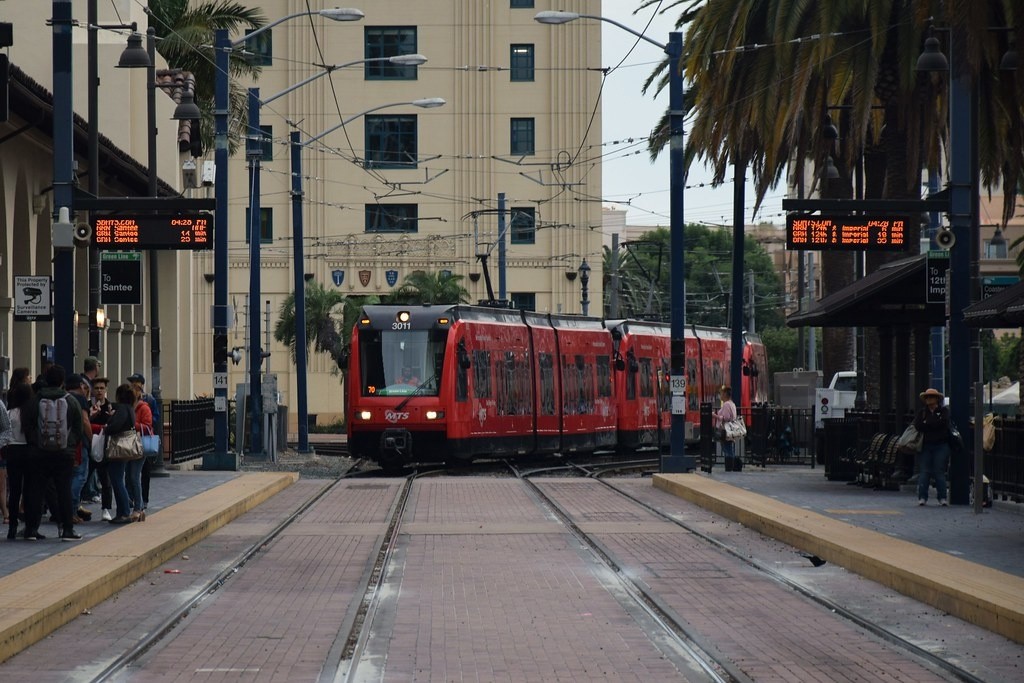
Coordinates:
39 391 72 452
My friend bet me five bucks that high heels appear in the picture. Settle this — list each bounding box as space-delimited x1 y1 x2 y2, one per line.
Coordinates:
130 509 146 522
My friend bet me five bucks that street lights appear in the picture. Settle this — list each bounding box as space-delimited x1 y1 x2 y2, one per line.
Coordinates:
205 8 365 471
290 97 447 454
535 10 697 473
247 54 427 453
911 23 1008 417
88 0 154 358
578 257 591 317
822 104 886 412
147 26 204 478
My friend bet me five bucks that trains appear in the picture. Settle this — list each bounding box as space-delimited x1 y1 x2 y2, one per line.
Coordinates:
346 298 771 472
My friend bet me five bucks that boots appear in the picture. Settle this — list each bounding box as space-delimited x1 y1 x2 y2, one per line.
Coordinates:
724 456 734 472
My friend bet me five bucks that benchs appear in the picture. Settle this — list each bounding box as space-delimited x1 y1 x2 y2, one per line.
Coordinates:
839 432 901 492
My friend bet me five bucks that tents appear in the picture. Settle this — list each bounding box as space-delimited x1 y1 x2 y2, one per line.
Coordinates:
986 382 1020 404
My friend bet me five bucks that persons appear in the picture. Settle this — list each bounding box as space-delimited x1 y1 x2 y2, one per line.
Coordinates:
915 388 952 507
0 356 159 542
396 365 419 386
712 386 736 471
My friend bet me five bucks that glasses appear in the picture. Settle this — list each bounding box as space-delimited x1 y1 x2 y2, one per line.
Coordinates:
96 363 101 367
924 394 937 399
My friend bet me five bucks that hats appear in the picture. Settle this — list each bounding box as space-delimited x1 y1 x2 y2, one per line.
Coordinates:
919 389 944 404
127 373 145 384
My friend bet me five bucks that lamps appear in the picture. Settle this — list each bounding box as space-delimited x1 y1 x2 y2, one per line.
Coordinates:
304 273 314 282
566 272 577 281
204 273 213 282
469 273 481 282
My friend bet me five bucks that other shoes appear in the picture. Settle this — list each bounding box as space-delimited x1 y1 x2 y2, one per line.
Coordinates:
143 502 147 509
76 506 92 518
109 515 132 523
72 514 83 524
6 528 17 539
81 492 102 503
3 510 10 524
938 499 947 506
23 530 45 541
41 513 48 522
918 498 926 506
58 530 83 541
101 508 112 521
129 497 134 508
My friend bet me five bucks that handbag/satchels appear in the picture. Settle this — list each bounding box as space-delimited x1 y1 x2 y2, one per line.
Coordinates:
6 407 26 444
105 404 144 460
91 427 106 462
936 408 963 448
139 423 159 456
721 416 746 441
